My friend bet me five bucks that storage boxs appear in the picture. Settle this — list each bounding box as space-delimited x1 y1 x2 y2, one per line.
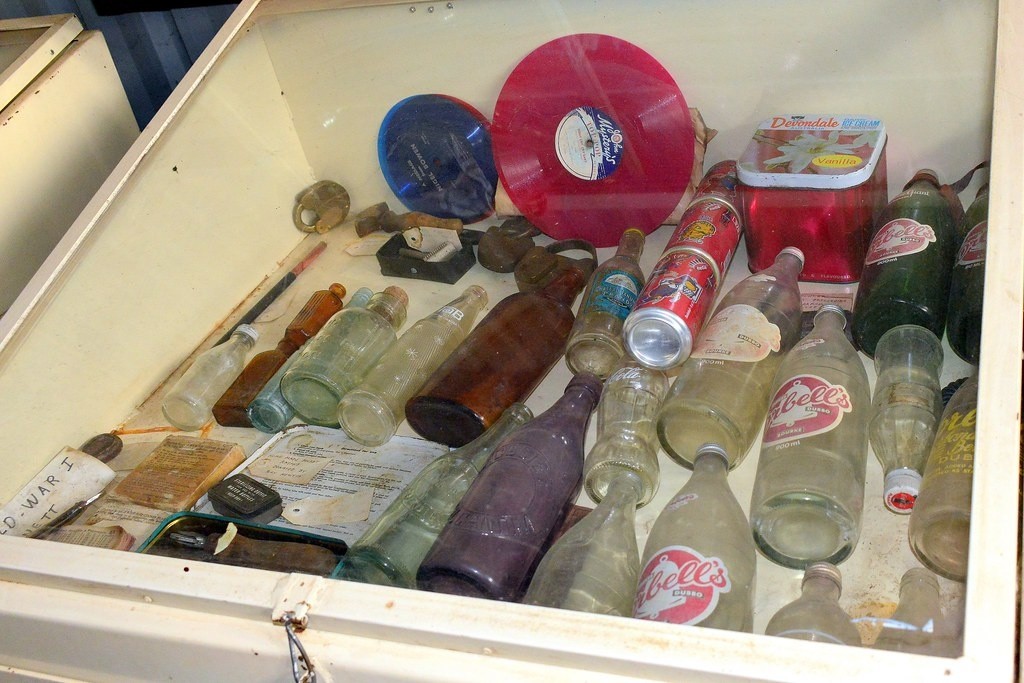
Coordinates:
0 0 1024 683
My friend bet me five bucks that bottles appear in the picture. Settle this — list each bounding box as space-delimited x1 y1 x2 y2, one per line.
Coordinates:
160 169 990 657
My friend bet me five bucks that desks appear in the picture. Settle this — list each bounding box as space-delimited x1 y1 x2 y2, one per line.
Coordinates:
0 12 140 316
